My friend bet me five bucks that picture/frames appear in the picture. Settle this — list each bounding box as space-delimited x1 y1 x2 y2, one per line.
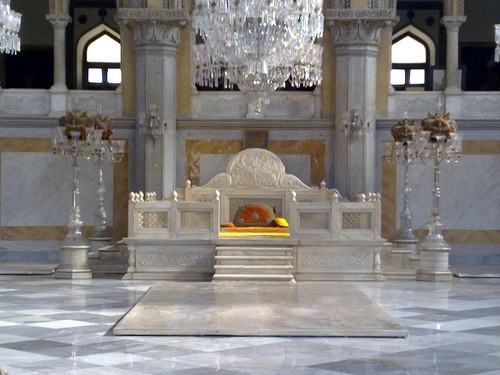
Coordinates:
429 65 467 91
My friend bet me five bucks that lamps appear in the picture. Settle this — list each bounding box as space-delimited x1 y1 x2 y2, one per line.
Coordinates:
192 0 324 113
342 109 372 140
417 96 462 248
0 0 21 56
138 101 167 144
384 110 421 240
53 104 126 246
